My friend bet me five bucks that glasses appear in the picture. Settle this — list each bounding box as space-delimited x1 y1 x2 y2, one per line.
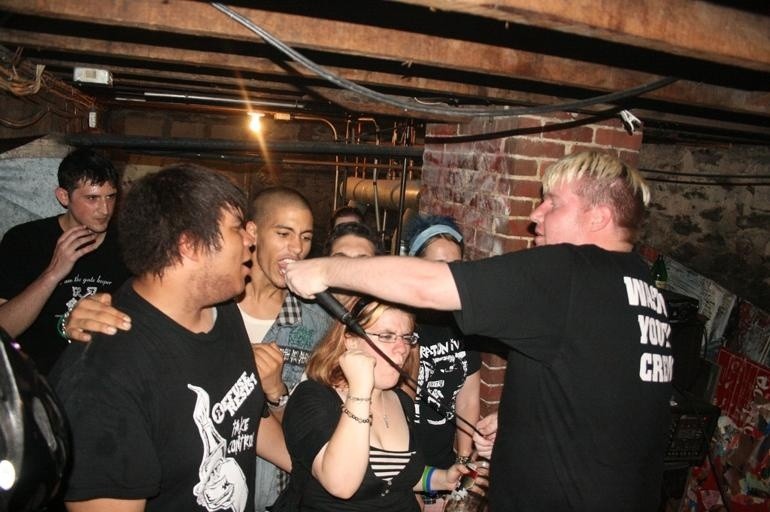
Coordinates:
366 331 421 345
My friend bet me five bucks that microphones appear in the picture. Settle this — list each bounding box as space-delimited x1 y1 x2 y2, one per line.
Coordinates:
316 292 351 324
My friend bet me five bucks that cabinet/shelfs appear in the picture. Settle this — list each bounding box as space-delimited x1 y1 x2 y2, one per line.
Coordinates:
663 422 769 512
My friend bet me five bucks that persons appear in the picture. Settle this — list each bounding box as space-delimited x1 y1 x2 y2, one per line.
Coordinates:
1 146 122 363
279 149 675 509
52 165 329 512
272 207 480 511
